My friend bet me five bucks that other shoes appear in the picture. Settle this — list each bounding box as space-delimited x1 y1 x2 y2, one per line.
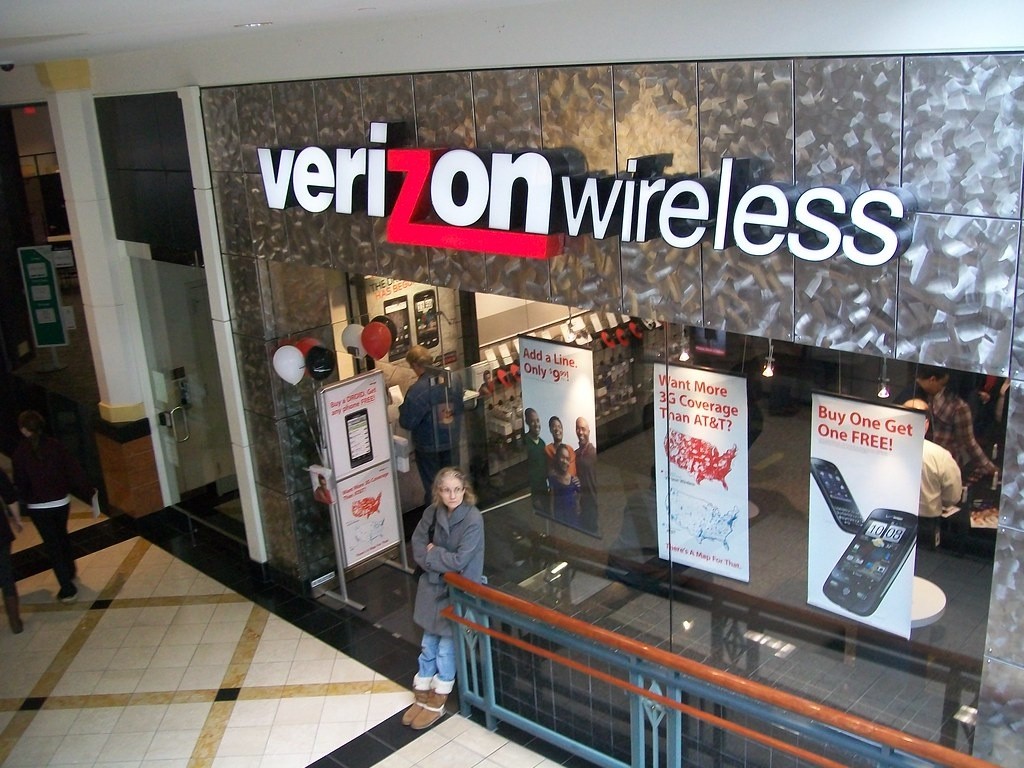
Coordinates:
58 585 77 602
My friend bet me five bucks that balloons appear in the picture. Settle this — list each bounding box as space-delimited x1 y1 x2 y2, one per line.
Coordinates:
370 316 397 344
306 346 336 381
273 345 306 386
361 322 391 361
341 324 368 359
292 337 323 381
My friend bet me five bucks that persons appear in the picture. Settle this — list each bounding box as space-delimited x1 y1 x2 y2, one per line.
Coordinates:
0 467 24 634
400 344 465 497
315 474 332 504
895 365 1010 482
524 407 599 534
904 398 964 547
608 465 657 569
11 409 97 600
480 370 496 399
402 467 488 730
642 400 764 453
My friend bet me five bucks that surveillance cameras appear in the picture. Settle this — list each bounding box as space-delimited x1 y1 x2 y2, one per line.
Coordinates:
0 61 15 72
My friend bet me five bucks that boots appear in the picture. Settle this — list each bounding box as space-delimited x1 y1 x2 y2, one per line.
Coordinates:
402 671 433 726
5 595 23 634
410 674 456 729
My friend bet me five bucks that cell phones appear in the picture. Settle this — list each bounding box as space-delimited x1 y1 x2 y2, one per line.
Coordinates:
809 456 864 534
384 295 413 361
823 508 917 617
345 408 373 468
414 290 439 349
